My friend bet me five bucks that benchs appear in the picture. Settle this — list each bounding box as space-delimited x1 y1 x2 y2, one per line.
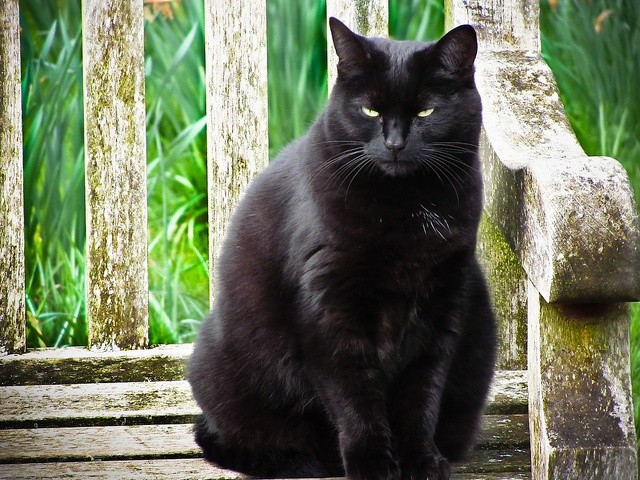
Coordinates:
1 0 640 478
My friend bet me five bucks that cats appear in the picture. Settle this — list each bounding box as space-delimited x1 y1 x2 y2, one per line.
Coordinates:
183 16 501 480
